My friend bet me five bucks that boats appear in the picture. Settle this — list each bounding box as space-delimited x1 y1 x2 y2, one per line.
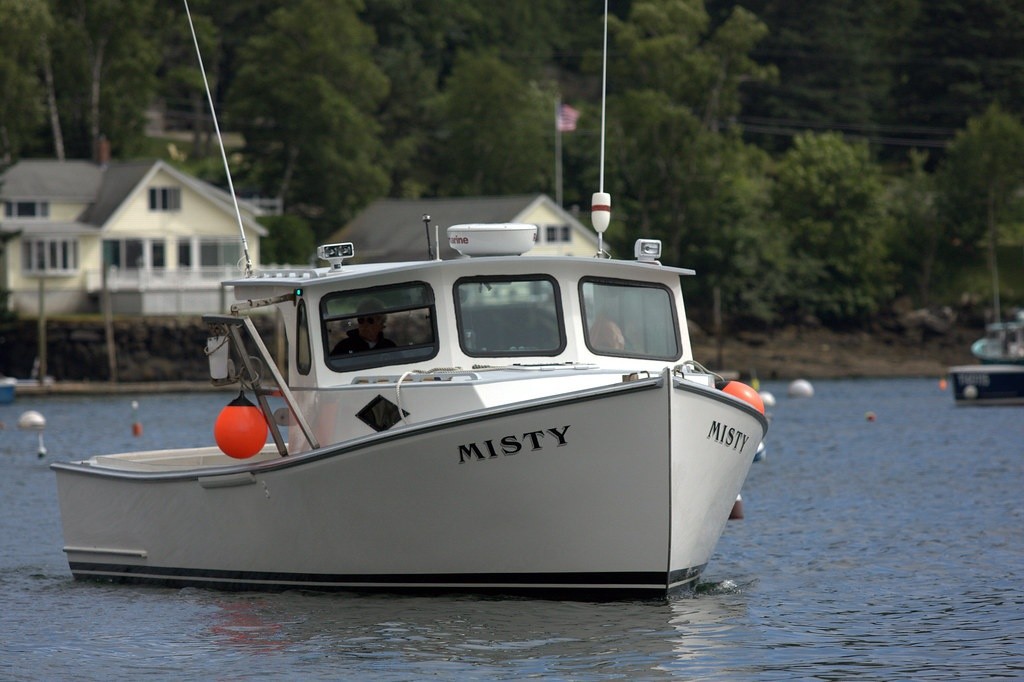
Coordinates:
948 320 1024 408
48 1 770 606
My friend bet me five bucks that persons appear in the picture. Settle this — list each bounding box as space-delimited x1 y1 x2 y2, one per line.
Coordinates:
329 299 406 370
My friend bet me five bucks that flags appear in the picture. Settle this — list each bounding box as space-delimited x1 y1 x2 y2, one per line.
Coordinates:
555 104 579 131
